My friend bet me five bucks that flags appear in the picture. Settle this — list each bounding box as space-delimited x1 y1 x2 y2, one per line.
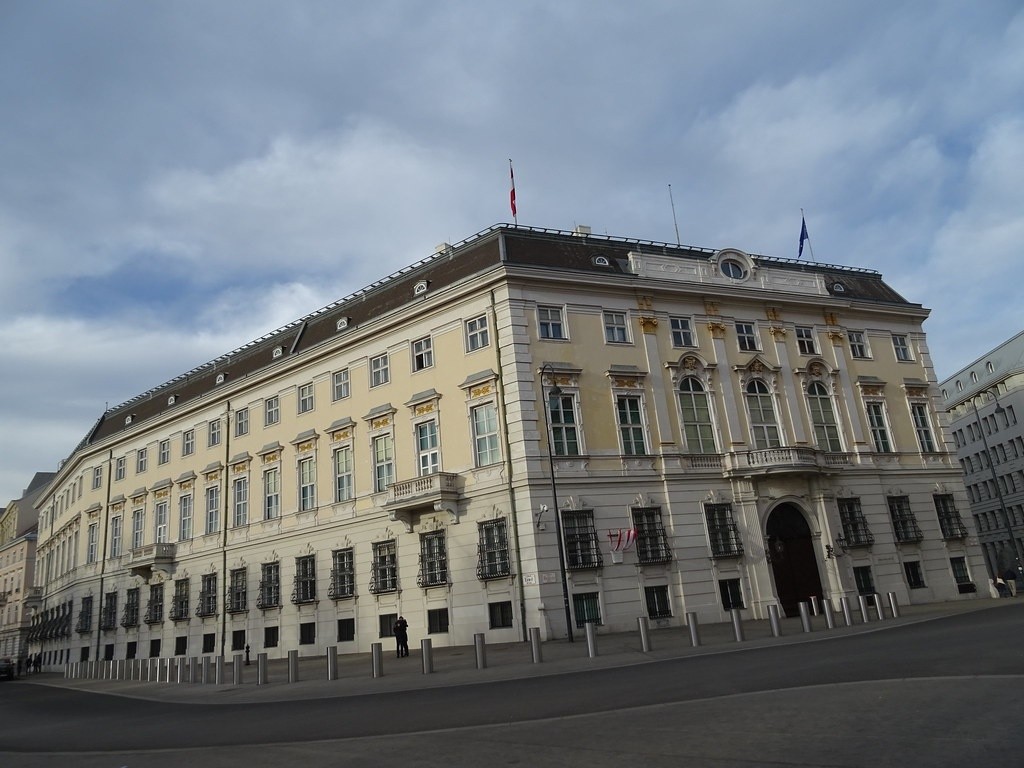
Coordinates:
509 165 517 218
607 528 637 551
798 218 810 259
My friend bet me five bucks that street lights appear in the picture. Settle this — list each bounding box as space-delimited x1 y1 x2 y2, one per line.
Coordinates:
94 446 115 660
219 398 232 656
539 361 575 643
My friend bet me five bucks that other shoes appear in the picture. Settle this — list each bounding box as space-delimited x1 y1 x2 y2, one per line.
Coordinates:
1012 596 1017 597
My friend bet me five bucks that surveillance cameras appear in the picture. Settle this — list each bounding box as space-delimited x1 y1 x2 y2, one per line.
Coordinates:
543 506 549 512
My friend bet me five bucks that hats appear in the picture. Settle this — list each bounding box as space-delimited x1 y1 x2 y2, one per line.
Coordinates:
1010 567 1014 569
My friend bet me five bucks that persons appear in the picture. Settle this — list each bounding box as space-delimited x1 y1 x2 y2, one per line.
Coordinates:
16 658 23 677
24 654 33 676
393 616 409 658
34 653 42 673
